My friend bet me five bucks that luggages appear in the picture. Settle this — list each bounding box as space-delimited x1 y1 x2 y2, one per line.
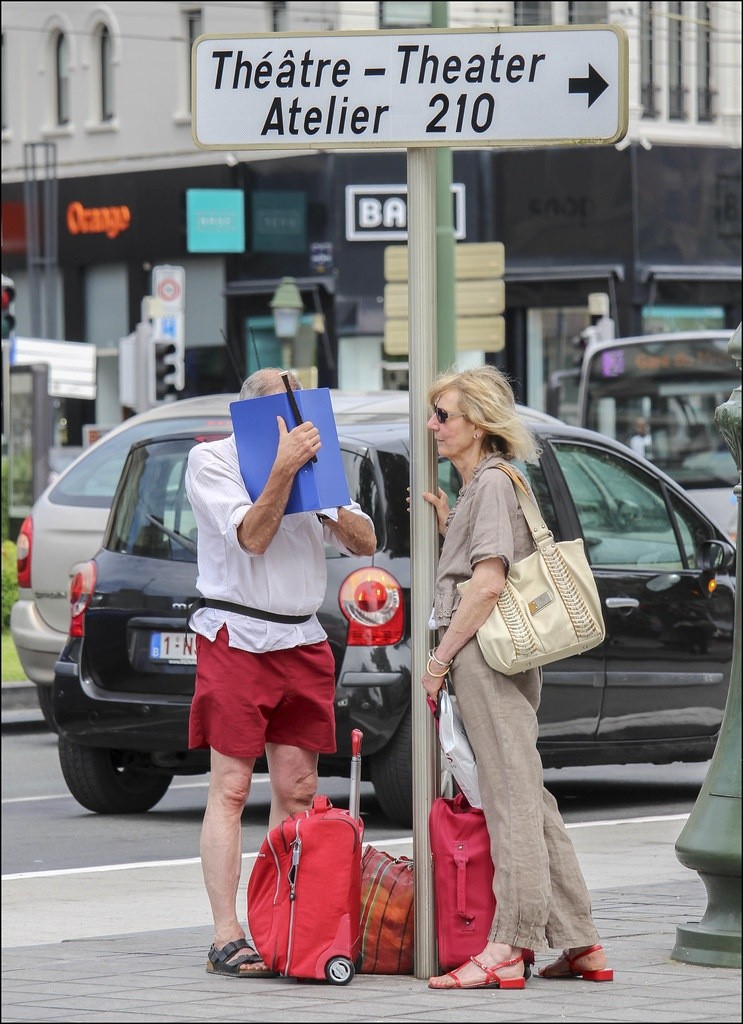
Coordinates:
429 792 536 980
246 728 363 985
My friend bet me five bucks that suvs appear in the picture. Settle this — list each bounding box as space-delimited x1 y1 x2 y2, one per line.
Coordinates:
10 387 737 742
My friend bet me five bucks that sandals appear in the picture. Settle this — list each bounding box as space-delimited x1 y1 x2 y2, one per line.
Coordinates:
532 945 613 982
206 938 279 979
428 955 525 990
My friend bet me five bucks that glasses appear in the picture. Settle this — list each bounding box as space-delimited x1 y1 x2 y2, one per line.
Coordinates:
433 403 468 424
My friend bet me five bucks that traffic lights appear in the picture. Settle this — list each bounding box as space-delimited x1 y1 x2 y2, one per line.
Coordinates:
1 274 15 347
146 338 178 408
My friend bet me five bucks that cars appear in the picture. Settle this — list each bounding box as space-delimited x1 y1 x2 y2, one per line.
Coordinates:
49 422 736 817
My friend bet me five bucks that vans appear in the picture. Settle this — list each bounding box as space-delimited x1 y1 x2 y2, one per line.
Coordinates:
571 331 743 555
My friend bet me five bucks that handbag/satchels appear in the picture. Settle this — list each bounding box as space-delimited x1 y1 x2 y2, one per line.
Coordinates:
439 689 484 809
359 844 414 975
456 462 607 676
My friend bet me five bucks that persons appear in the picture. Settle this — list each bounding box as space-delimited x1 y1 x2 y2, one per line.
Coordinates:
410 367 618 991
630 416 654 463
181 365 379 977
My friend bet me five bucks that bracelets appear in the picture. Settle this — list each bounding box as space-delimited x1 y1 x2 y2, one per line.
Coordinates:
426 645 454 677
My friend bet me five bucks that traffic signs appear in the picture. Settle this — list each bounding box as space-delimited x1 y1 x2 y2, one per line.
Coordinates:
190 21 631 154
